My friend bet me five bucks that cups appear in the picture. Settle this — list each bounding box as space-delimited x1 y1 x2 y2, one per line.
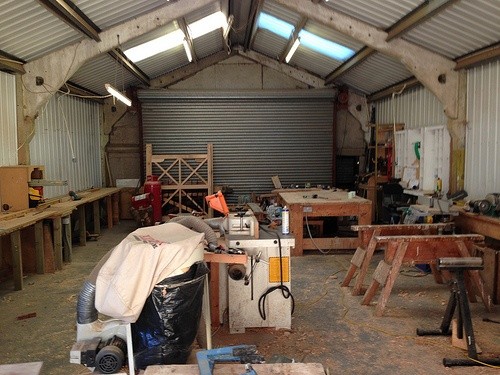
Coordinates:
348 192 355 199
29 194 38 208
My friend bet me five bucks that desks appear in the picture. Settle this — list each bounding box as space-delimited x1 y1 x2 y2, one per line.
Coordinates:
142 362 329 375
0 186 123 292
457 208 500 242
273 186 372 256
228 224 296 334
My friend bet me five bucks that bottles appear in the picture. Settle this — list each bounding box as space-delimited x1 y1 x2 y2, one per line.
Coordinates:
468 199 491 212
281 205 289 234
442 217 453 235
31 167 44 197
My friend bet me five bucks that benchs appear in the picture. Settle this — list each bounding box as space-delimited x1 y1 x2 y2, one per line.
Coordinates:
340 223 451 294
356 235 493 316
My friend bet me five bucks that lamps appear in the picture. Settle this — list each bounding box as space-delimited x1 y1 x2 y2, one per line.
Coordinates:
222 14 237 41
104 83 132 107
182 36 194 64
284 36 302 64
